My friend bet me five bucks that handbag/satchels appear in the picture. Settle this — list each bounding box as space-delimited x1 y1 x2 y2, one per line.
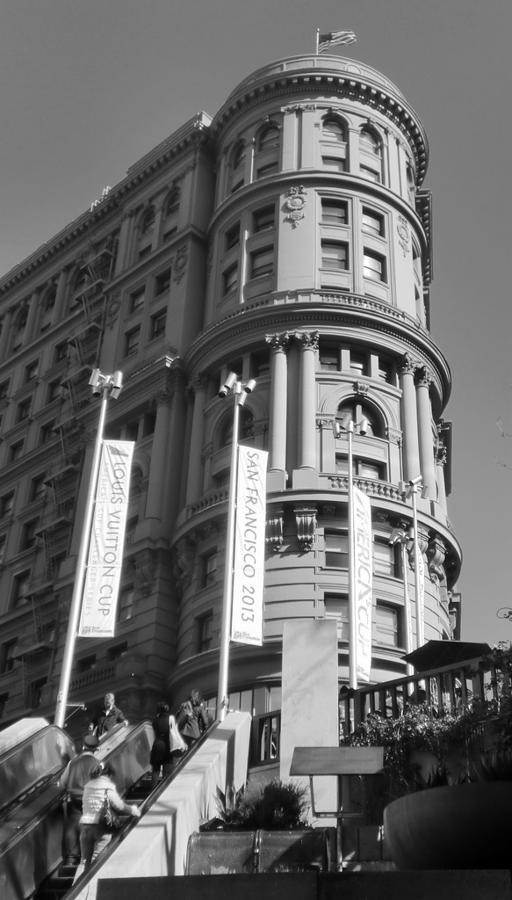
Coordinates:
99 805 122 830
176 707 193 731
168 727 188 757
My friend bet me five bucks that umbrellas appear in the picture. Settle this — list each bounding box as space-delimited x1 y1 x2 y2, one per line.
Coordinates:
397 635 500 683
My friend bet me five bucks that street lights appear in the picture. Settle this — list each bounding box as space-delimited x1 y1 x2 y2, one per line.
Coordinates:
214 368 258 725
331 418 370 689
398 475 424 649
387 526 416 697
53 368 125 733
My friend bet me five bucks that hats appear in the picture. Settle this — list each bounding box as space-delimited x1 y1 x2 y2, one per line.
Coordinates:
84 734 99 747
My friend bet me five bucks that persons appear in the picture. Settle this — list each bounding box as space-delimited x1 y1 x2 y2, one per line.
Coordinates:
150 698 186 787
84 691 129 742
56 734 108 867
73 761 140 888
176 687 211 746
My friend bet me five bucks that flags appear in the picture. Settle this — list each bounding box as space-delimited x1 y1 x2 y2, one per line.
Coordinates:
317 28 358 53
